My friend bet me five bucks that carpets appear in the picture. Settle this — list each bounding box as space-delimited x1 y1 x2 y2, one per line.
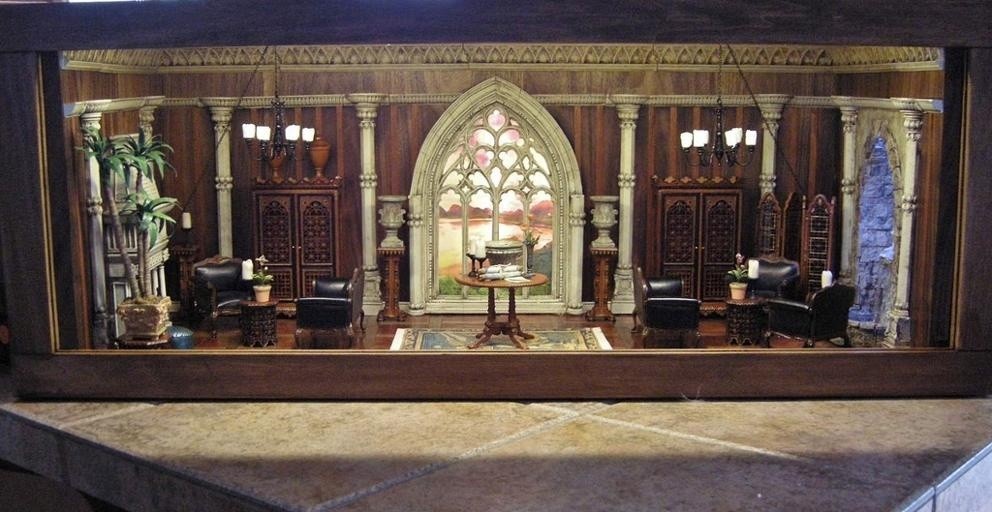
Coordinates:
387 327 615 352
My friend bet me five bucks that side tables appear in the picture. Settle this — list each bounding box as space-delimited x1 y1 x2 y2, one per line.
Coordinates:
171 241 204 310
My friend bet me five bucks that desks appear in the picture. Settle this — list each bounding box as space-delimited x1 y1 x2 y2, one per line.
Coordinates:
455 271 550 351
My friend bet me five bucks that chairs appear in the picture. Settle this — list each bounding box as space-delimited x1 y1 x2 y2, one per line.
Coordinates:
724 255 801 335
293 265 365 351
765 281 857 351
632 266 702 350
188 256 259 336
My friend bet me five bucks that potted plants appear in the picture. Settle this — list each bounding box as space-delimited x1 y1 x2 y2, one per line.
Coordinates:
70 120 184 340
252 272 274 303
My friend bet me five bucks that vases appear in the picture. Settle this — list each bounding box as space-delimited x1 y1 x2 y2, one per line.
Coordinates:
729 282 748 302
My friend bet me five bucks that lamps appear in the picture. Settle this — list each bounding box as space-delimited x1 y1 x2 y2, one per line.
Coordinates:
239 41 317 162
678 38 758 167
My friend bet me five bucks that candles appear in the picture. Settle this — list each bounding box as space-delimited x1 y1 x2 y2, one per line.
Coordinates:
473 241 486 257
241 258 255 282
821 270 834 291
467 236 477 257
179 209 192 228
748 259 759 280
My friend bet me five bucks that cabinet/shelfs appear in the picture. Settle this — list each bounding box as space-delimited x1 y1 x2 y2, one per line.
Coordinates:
250 176 345 321
649 183 745 320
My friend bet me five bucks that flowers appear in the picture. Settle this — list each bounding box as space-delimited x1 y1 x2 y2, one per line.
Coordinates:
728 252 748 282
